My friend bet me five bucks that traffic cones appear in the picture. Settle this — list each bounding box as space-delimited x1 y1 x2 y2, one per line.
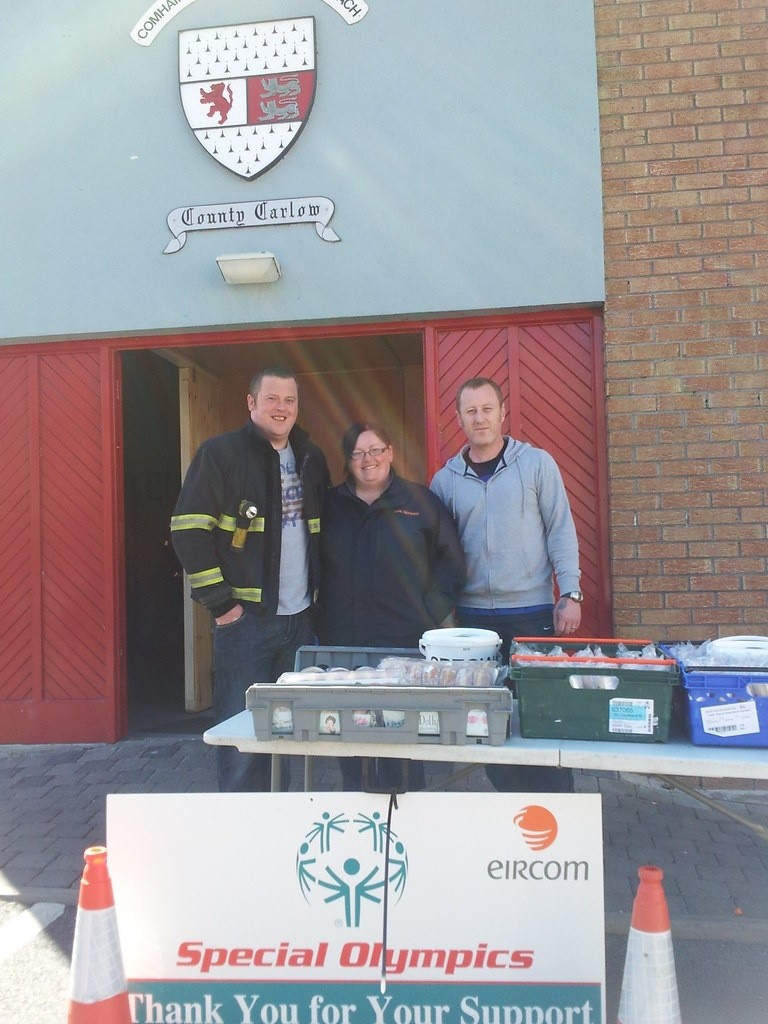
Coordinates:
615 862 684 1024
66 845 132 1024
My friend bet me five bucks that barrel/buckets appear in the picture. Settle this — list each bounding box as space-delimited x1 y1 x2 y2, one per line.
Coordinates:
419 628 503 662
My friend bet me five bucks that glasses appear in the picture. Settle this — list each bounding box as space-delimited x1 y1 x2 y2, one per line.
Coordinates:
349 444 388 461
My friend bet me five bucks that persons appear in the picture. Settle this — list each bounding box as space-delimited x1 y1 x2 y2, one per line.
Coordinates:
429 376 584 792
168 360 335 792
310 422 468 793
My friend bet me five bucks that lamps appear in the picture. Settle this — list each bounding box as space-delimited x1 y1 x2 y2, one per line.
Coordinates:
216 250 281 287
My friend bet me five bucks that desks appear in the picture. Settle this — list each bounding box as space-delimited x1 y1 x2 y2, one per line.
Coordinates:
202 705 767 832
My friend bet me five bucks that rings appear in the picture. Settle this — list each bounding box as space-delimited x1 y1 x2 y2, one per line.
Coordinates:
571 627 576 629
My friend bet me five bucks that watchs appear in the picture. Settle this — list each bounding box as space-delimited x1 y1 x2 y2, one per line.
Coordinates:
561 590 584 603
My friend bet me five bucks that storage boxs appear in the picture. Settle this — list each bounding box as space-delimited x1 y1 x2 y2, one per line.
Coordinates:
509 634 678 742
656 639 767 748
